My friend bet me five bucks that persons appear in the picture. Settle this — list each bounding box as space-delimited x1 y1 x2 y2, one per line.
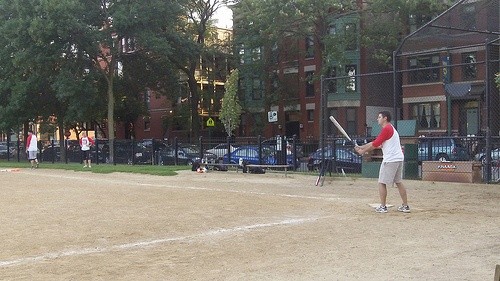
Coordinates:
25 130 39 169
354 111 410 213
79 132 95 168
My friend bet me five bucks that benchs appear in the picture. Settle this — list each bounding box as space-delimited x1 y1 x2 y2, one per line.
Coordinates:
245 164 294 178
207 164 240 173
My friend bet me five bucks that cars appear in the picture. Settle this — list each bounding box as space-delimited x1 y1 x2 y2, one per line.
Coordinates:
259 136 295 155
223 145 301 168
307 146 362 173
206 143 241 159
475 148 500 166
0 138 217 166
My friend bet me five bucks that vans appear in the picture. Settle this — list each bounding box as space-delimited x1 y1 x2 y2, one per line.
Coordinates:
417 137 460 161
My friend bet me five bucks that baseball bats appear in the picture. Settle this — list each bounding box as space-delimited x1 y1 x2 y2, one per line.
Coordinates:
329 116 364 157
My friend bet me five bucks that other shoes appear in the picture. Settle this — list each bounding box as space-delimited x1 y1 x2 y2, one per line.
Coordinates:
375 205 388 213
398 204 410 212
